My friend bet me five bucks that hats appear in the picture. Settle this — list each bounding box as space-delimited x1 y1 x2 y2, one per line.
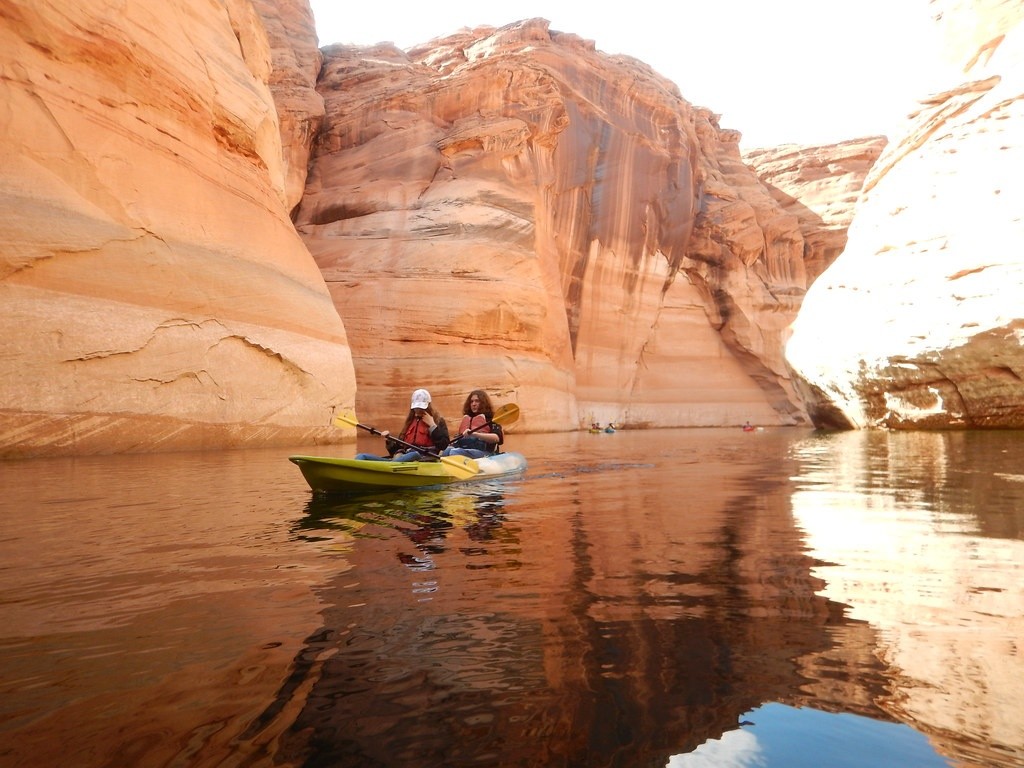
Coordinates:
411 389 432 410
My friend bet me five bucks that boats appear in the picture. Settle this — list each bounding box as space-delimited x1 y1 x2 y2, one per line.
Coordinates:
288 452 528 493
744 427 754 431
590 428 616 434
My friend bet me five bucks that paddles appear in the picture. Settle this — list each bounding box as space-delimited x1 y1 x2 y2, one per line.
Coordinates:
434 403 521 443
336 413 481 481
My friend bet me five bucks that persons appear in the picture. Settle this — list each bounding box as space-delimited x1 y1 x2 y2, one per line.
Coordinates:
609 422 615 429
441 390 504 459
592 423 601 429
744 421 752 428
355 389 450 462
